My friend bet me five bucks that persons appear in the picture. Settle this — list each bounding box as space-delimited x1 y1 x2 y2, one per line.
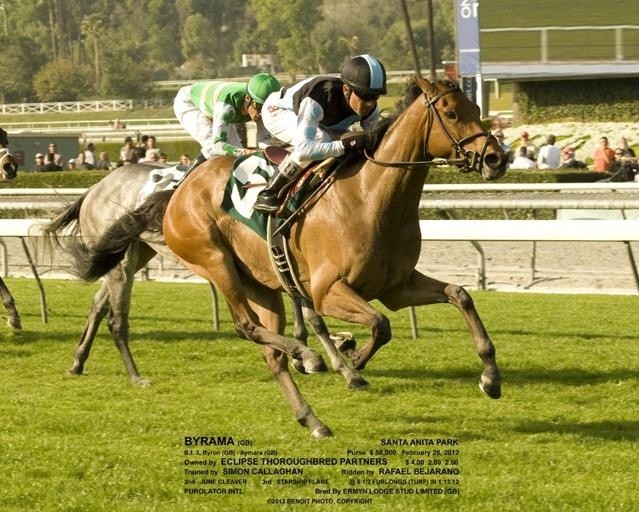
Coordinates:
180 153 190 164
250 54 387 213
592 136 616 172
76 151 92 170
113 118 125 129
31 152 44 171
173 70 280 189
510 146 536 170
119 134 168 166
558 147 589 170
608 147 634 182
495 131 514 170
68 158 77 171
96 151 112 170
617 136 636 157
38 153 62 171
44 142 63 166
537 134 561 170
515 130 538 160
84 143 95 165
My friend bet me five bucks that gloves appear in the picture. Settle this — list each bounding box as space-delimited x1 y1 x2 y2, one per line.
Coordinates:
343 134 366 152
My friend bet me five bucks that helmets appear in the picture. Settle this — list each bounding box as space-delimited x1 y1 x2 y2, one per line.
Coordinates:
246 72 280 105
341 53 388 95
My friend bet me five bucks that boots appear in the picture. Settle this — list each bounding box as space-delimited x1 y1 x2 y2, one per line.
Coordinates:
252 152 303 212
173 152 208 189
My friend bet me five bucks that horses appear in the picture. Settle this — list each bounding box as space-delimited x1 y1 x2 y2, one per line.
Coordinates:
0 127 22 329
24 160 370 386
58 77 509 440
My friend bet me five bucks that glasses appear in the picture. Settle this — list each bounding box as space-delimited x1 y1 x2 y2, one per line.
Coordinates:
354 89 380 101
251 101 263 111
